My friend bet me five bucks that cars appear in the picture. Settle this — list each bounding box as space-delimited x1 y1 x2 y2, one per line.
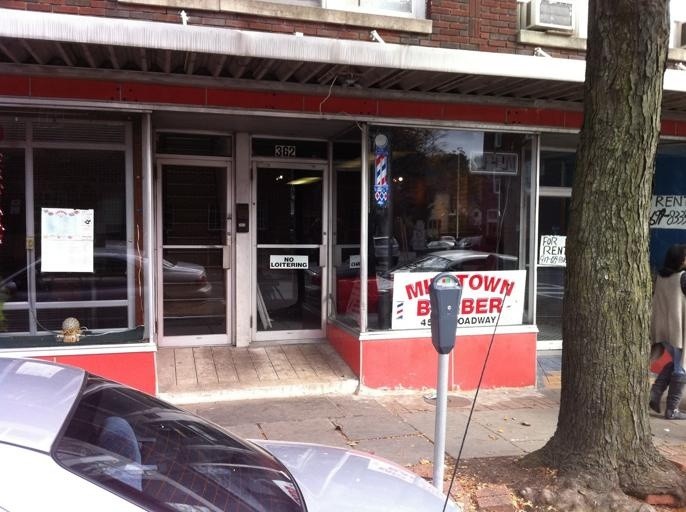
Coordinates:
302 253 376 321
0 356 465 512
421 234 485 252
0 244 211 328
375 250 521 290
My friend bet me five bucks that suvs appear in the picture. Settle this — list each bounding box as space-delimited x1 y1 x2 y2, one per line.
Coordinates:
372 236 401 267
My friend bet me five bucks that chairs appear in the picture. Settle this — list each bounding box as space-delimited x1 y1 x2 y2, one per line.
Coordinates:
257 252 372 320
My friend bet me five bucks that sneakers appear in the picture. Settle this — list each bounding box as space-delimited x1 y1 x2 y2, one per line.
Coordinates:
649 399 662 413
664 408 686 420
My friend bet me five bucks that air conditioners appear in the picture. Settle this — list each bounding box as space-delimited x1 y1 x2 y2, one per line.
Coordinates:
523 0 576 35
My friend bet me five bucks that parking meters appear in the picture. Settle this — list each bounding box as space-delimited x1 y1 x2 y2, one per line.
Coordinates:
429 272 462 493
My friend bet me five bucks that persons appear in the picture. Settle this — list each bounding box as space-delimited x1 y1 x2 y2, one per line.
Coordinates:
648 243 685 421
